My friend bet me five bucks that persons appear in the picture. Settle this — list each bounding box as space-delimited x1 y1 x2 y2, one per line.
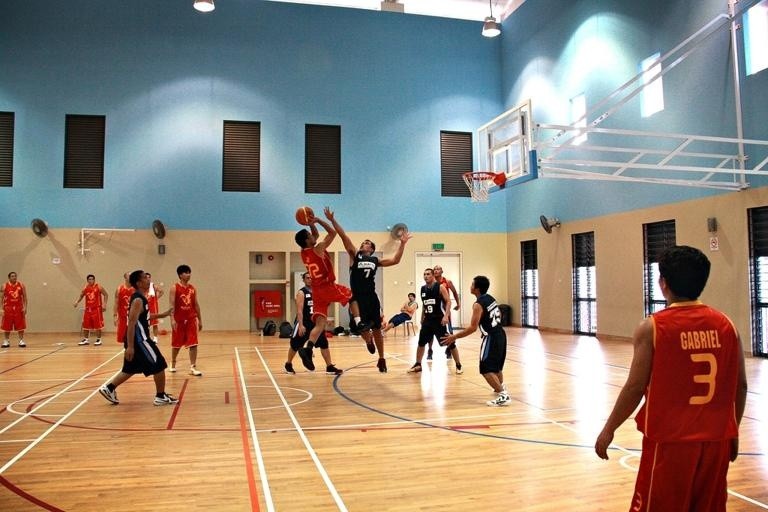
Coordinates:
283 206 512 407
71 274 109 347
0 269 29 349
593 243 748 511
99 265 202 405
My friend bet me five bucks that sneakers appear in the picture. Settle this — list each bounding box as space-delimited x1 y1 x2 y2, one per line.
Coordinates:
18 340 25 346
170 367 177 372
189 368 201 374
2 339 10 346
153 393 179 406
376 358 387 373
410 348 463 375
99 386 119 404
298 347 316 371
285 361 296 376
94 338 101 345
487 393 512 407
78 338 89 345
326 364 344 374
366 336 376 353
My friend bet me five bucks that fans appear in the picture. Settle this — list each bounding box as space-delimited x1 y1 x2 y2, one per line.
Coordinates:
30 218 47 238
387 222 410 241
539 214 562 235
152 219 167 239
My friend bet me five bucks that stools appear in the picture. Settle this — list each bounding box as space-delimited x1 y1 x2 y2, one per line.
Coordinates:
395 317 416 335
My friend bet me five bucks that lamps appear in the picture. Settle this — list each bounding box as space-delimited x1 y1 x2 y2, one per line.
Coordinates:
191 0 215 12
479 0 501 40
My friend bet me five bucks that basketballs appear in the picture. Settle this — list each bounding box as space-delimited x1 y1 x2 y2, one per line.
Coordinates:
296 206 314 226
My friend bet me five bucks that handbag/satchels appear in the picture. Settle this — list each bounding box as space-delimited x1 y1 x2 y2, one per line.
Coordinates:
262 319 350 338
